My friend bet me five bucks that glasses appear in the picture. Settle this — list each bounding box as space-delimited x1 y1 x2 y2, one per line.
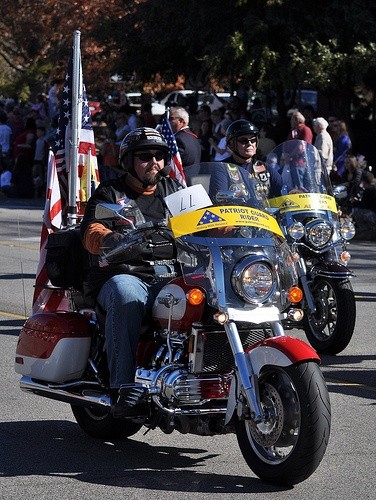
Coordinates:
235 137 256 145
134 151 165 162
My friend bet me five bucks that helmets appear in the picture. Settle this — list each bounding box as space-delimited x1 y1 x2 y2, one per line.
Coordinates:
119 127 168 165
225 120 259 146
197 105 211 113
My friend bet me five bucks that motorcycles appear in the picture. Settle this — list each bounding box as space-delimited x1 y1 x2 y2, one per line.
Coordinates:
214 139 357 355
15 166 331 486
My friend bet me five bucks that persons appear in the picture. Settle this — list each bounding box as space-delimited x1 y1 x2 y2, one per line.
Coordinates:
0 77 376 243
79 126 251 417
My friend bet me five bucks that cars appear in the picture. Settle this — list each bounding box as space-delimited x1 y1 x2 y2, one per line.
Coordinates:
125 92 157 115
295 89 319 111
150 89 278 123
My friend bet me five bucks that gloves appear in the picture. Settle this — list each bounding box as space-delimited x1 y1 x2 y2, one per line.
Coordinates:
102 231 132 255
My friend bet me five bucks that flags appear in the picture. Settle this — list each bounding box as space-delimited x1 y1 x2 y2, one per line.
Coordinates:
159 116 187 189
32 42 100 313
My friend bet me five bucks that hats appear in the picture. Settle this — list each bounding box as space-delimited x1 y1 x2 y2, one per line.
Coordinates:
313 118 328 129
